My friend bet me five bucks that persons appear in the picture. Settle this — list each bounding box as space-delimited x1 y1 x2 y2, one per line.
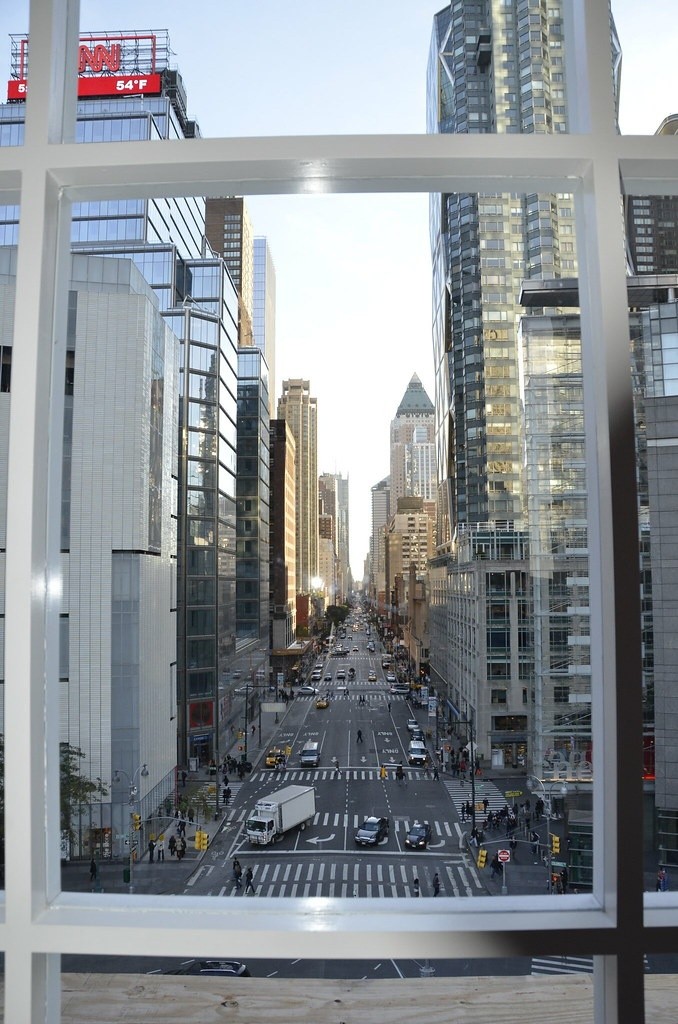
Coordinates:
233 858 257 894
433 873 440 896
656 868 669 892
414 879 419 896
149 795 194 863
356 730 363 742
90 859 97 879
381 760 406 783
281 676 304 702
252 724 255 735
388 703 391 712
326 689 365 705
182 770 187 786
335 762 342 775
461 797 567 894
433 729 480 781
223 754 242 805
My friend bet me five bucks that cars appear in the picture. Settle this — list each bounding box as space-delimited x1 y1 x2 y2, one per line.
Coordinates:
355 816 389 847
405 823 432 849
406 719 424 741
311 663 345 681
265 746 282 767
366 638 375 652
368 670 376 681
316 698 329 709
386 670 396 681
340 605 370 639
330 643 358 658
298 687 319 695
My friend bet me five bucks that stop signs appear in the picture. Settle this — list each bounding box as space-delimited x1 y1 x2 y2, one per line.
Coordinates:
497 849 510 863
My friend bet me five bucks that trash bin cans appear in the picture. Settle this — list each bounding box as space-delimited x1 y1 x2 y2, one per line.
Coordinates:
476 830 483 842
245 761 252 773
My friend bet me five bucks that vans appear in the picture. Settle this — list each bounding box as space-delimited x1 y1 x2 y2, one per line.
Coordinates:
299 742 321 768
390 683 410 695
407 741 427 766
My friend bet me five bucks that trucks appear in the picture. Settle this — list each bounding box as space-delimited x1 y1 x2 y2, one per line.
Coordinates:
246 784 315 845
382 652 392 669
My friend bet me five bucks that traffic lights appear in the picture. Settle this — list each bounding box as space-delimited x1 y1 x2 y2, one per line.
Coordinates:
133 813 142 831
201 832 209 850
553 875 560 883
238 732 242 739
285 745 291 755
477 850 488 869
553 836 560 854
238 745 242 751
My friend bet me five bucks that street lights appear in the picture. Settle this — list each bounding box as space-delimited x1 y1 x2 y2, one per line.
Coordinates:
112 763 149 894
526 775 567 894
245 684 275 761
